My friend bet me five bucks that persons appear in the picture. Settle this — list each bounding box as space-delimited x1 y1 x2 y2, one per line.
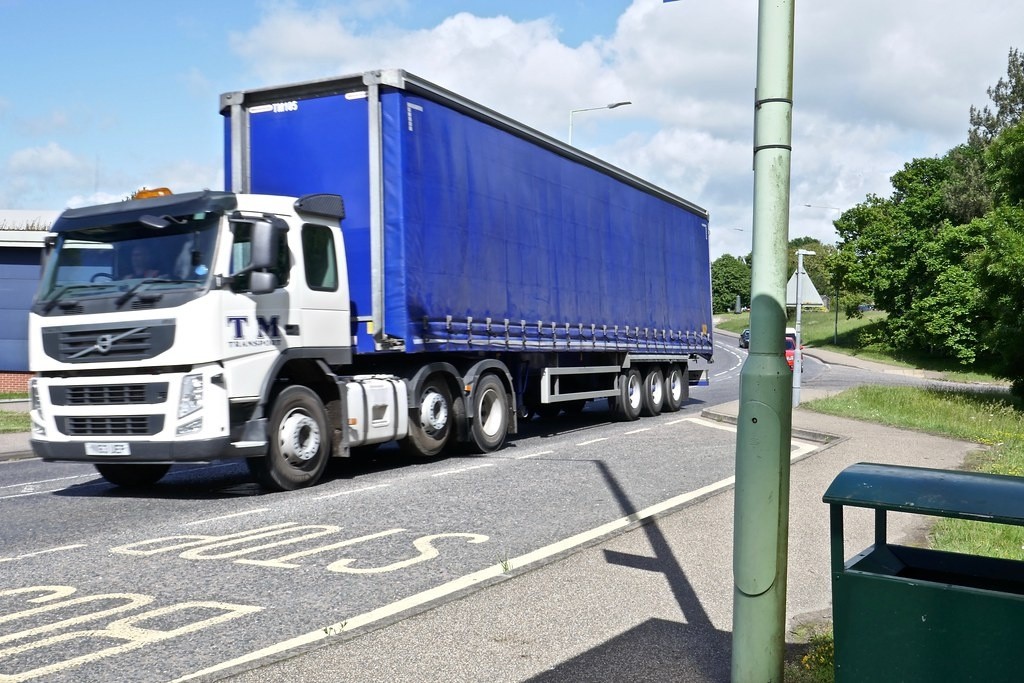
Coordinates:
120 245 162 280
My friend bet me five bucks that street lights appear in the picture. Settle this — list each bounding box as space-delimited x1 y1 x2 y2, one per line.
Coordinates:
568 101 632 145
791 251 816 409
805 204 841 345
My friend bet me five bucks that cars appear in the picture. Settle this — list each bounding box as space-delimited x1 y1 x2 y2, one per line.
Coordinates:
785 327 802 346
738 330 750 349
785 337 805 374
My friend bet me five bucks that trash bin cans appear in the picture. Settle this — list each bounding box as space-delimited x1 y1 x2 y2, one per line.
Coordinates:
822 462 1024 683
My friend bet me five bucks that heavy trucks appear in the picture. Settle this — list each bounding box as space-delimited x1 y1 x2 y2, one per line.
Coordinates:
28 66 715 491
